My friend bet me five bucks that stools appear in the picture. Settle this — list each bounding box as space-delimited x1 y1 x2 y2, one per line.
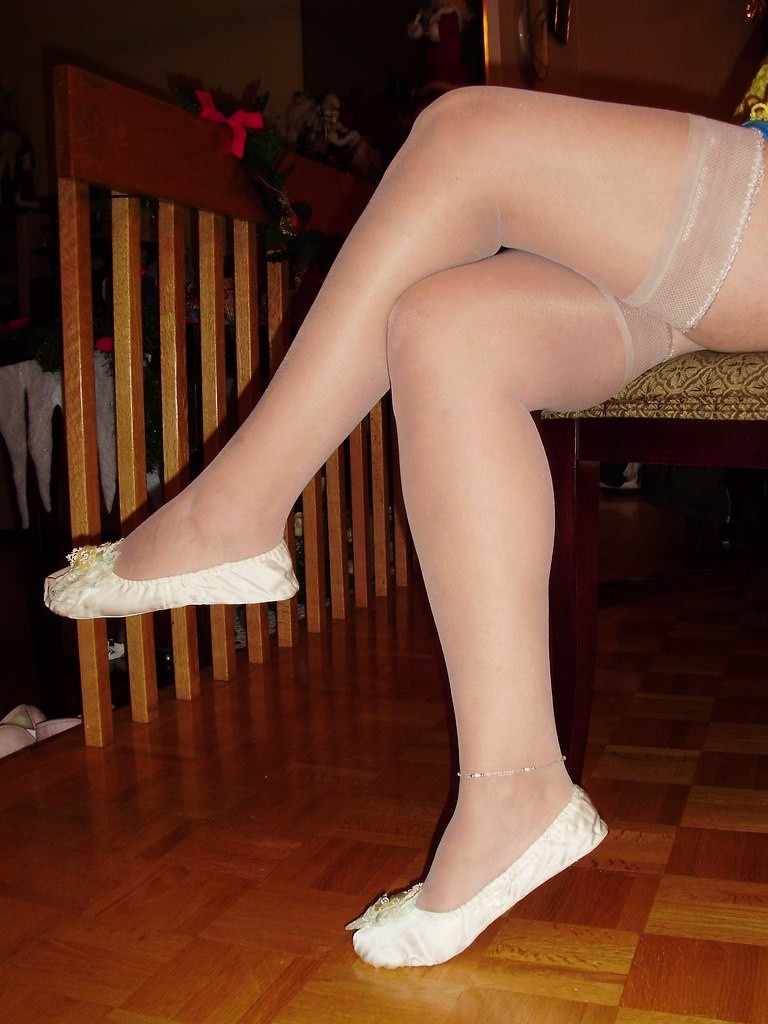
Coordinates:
544 352 768 783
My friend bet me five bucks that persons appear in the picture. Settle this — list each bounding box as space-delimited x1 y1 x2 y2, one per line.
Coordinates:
38 54 768 975
